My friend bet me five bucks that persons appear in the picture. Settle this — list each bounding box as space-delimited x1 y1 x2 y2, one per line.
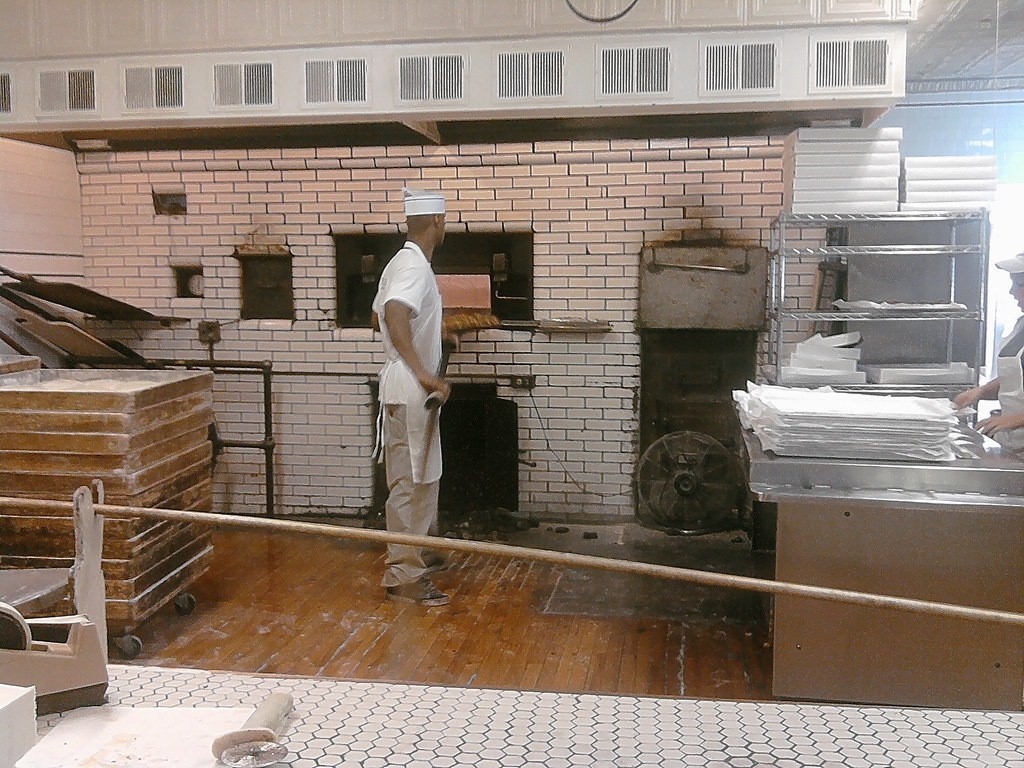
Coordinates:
953 254 1024 452
369 195 450 607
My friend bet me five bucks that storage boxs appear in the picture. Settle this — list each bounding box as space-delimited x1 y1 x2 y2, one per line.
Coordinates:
778 330 976 385
781 128 998 215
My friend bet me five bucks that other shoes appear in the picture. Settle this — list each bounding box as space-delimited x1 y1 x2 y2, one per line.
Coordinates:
419 550 445 573
386 575 450 605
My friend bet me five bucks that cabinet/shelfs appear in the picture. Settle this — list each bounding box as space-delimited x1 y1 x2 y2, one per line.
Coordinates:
767 209 989 430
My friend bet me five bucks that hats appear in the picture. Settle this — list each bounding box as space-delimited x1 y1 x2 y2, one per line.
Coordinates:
402 186 446 216
994 251 1024 273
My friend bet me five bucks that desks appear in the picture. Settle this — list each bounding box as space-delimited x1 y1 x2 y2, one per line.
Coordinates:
35 662 1024 768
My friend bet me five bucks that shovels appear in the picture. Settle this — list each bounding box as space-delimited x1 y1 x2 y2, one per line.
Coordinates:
0 264 161 369
415 312 500 487
